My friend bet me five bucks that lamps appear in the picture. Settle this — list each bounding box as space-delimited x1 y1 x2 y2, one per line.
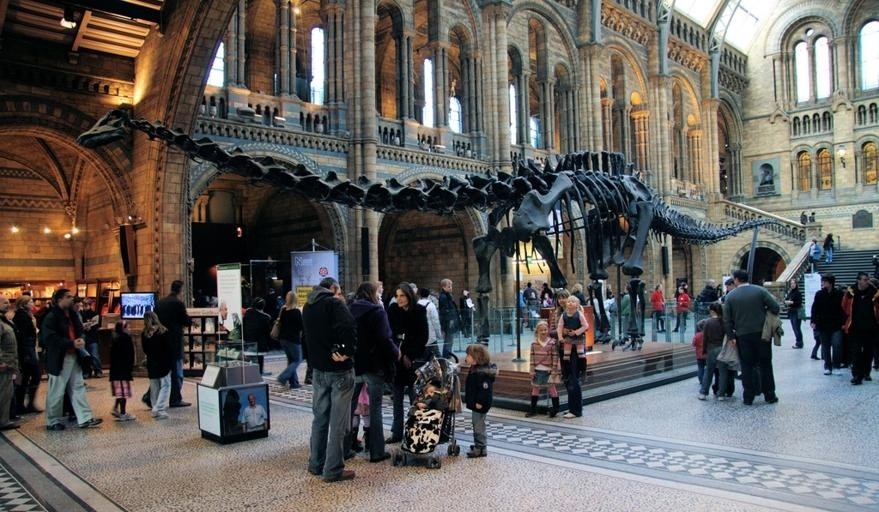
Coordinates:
837 144 847 169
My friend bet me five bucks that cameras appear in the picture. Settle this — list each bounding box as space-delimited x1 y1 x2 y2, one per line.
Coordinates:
331 343 349 356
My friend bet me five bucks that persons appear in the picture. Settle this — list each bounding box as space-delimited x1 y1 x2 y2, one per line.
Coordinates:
801 211 808 225
807 239 822 271
872 254 879 280
823 233 836 264
809 211 816 223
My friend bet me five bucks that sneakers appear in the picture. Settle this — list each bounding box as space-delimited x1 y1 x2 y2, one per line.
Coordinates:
1 406 43 431
465 445 490 458
78 417 106 428
117 411 140 423
46 420 68 432
697 393 778 406
850 375 874 385
823 368 832 375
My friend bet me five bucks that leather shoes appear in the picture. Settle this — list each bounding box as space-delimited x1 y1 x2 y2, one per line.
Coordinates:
321 465 357 483
384 437 402 444
143 395 152 409
370 452 392 463
172 399 196 409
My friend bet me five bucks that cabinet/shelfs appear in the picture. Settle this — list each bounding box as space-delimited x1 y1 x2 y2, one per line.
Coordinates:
75 278 98 302
96 278 121 315
182 315 219 377
0 280 67 312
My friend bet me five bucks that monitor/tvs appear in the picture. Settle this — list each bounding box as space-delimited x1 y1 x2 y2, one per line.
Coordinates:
120 292 157 320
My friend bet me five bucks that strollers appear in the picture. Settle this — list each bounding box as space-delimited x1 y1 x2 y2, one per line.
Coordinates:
391 350 464 469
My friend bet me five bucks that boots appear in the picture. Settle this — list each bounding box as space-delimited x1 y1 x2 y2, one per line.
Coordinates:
545 395 563 418
522 395 540 419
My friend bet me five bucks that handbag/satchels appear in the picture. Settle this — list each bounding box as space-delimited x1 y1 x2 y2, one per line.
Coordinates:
797 308 807 320
269 307 283 340
